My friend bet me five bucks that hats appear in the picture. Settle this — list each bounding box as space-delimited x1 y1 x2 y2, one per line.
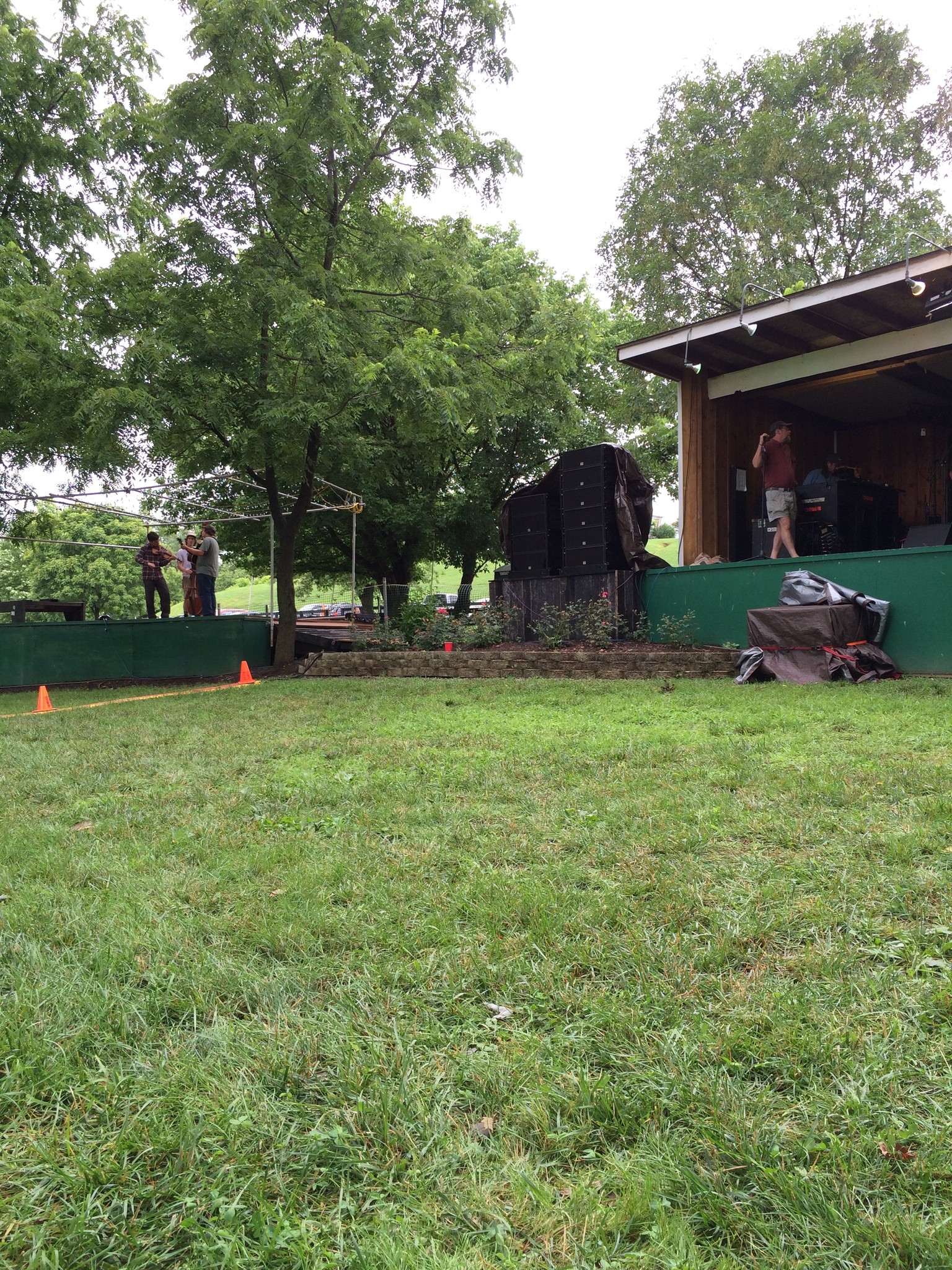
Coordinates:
186 532 197 538
770 420 793 431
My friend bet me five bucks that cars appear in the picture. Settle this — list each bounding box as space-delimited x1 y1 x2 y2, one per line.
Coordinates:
215 608 280 619
408 593 458 614
296 602 362 618
468 597 490 613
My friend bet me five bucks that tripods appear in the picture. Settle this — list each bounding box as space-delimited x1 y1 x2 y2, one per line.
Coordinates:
739 438 774 563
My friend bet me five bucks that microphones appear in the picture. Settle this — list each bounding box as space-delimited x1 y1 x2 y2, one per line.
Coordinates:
763 436 770 442
941 461 947 466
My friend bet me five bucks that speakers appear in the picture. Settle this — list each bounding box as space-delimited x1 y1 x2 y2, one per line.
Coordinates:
902 522 952 549
506 444 631 579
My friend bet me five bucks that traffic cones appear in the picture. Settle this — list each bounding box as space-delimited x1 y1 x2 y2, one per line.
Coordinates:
238 661 257 684
32 686 58 713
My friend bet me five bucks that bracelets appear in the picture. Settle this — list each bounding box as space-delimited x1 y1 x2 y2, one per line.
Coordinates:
186 546 188 550
758 443 762 446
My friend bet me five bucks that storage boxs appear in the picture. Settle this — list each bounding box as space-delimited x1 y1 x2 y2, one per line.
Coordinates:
511 493 563 577
561 444 631 570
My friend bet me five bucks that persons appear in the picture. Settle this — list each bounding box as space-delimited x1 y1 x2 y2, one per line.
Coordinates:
135 524 219 619
753 422 799 558
802 453 840 486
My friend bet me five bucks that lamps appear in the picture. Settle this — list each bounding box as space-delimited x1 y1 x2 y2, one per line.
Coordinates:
905 233 951 296
684 328 701 374
740 283 791 335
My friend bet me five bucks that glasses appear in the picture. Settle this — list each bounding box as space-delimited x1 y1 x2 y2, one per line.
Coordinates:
782 427 790 431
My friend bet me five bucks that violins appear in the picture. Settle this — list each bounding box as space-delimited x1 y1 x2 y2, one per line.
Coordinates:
152 544 183 563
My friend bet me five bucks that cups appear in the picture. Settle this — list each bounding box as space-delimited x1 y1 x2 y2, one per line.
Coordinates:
444 642 453 652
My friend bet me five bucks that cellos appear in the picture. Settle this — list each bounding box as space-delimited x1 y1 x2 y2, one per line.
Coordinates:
184 570 203 616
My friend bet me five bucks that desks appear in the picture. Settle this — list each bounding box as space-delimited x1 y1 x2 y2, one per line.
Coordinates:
0 601 86 624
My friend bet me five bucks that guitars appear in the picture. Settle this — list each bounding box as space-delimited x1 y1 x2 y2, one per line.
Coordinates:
176 537 200 571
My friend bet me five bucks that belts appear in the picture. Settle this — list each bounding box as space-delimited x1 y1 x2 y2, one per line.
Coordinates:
766 487 793 492
